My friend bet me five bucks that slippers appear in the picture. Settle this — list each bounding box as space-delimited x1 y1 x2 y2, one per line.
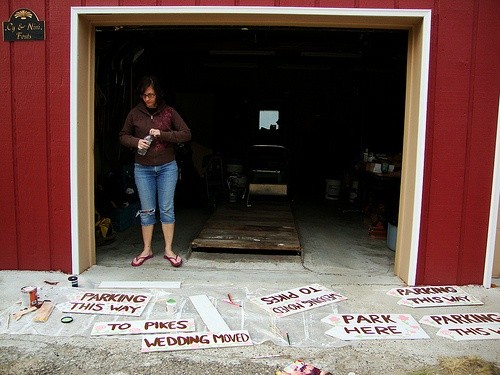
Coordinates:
131 251 182 267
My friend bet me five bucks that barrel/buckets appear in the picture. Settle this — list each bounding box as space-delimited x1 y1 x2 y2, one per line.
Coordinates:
21 286 38 308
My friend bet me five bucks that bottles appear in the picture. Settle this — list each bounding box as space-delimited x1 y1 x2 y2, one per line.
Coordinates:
137 135 153 155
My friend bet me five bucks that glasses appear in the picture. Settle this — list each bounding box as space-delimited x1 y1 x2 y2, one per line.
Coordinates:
139 93 157 98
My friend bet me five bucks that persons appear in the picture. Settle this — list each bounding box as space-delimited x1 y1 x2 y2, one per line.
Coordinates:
118 78 191 267
